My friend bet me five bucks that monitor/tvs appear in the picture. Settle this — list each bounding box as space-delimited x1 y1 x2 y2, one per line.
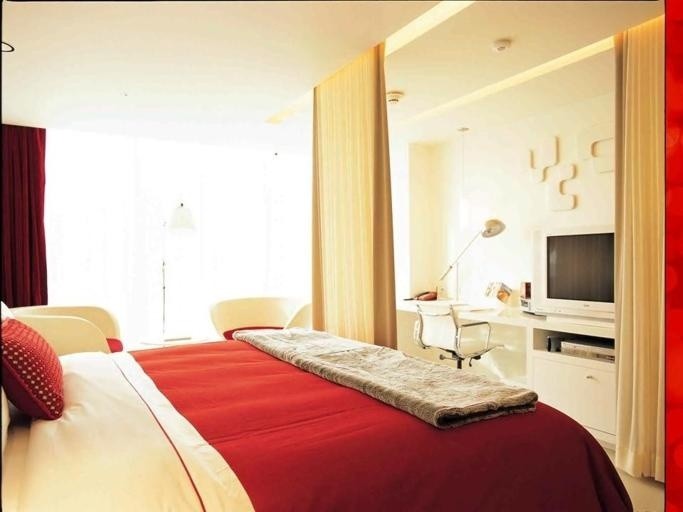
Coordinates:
532 224 614 322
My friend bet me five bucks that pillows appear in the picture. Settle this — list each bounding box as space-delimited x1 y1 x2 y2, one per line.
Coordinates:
0 317 64 419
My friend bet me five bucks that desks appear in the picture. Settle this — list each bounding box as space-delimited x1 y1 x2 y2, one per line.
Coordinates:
398 285 616 339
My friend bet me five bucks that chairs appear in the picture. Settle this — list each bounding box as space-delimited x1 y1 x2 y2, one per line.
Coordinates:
414 304 502 369
212 298 284 339
9 305 125 351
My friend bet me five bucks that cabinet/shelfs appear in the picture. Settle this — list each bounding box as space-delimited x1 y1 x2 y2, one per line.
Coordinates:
525 328 620 458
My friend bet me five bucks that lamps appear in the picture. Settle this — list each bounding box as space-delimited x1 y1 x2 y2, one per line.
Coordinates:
484 280 513 302
387 90 403 104
492 39 511 51
431 219 505 301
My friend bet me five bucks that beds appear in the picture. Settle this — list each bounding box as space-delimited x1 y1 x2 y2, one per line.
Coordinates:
0 331 518 512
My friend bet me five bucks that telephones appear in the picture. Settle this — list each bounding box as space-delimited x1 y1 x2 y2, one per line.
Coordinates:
404 290 437 301
